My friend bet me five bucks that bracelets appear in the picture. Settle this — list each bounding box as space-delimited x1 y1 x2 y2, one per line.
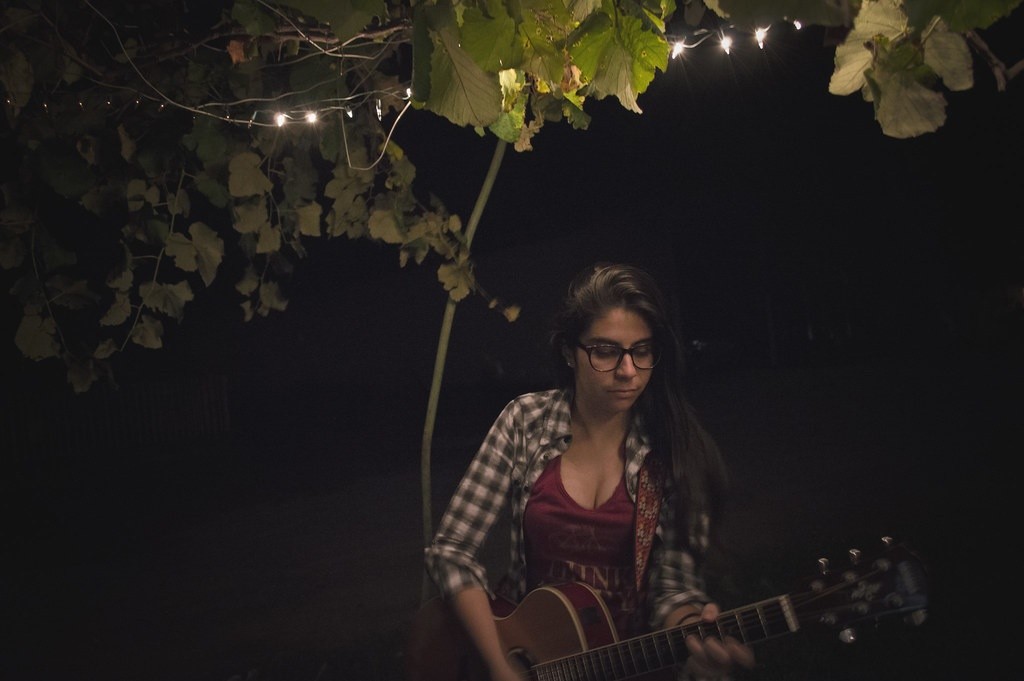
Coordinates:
675 611 702 626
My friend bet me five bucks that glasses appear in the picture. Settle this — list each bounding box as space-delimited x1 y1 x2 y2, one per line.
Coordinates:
574 338 662 372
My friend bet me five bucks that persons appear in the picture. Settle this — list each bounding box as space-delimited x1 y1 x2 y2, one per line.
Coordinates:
424 261 756 681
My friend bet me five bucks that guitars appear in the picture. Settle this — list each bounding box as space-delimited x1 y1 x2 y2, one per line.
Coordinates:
408 534 934 681
